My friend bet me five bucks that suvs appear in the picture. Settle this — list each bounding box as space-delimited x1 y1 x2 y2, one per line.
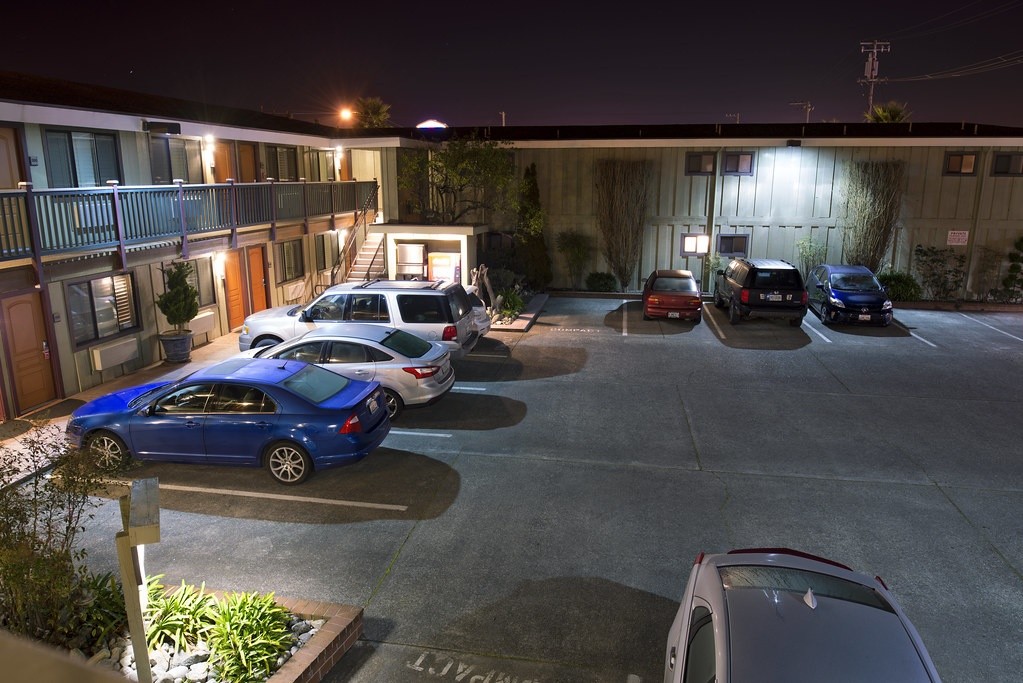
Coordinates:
713 258 808 327
67 286 117 341
235 279 480 374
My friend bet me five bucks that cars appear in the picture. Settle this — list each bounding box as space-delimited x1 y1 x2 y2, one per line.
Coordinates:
665 547 940 683
223 324 456 422
641 269 703 324
329 284 493 341
65 358 393 488
805 264 894 326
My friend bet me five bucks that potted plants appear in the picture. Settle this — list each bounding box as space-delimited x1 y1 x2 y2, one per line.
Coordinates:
153 261 201 359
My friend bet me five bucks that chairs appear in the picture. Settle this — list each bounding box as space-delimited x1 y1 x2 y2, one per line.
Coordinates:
679 282 689 289
354 297 383 319
223 388 264 412
